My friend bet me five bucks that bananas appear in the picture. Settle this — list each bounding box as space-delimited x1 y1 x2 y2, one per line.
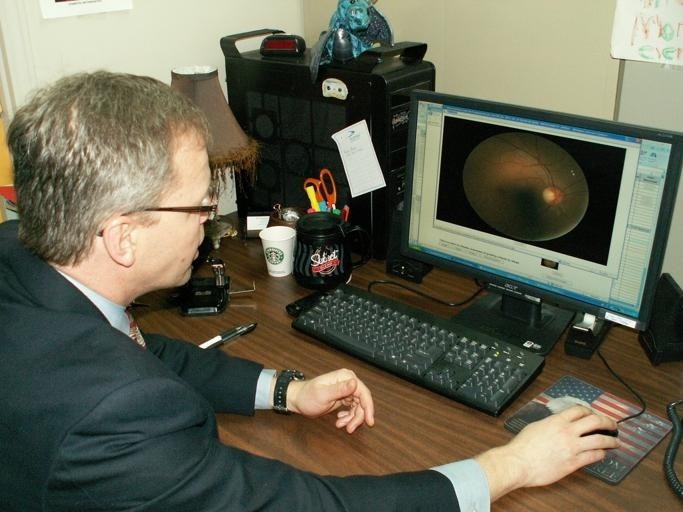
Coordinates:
292 283 546 416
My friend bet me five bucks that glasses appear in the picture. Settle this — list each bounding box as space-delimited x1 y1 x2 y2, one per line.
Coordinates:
168 65 260 249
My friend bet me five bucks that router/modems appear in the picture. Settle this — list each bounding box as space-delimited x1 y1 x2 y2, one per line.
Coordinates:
583 312 597 331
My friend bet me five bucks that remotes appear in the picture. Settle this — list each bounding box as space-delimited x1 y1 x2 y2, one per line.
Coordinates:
587 430 620 437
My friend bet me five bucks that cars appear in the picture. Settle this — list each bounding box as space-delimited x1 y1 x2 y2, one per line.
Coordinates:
259 226 296 277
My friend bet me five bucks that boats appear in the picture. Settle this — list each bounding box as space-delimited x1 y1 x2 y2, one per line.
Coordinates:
637 273 683 369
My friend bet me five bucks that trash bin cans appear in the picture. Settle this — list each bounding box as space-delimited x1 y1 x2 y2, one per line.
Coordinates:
273 368 305 415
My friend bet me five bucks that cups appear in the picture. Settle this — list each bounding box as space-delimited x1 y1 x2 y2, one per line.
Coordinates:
96 184 217 237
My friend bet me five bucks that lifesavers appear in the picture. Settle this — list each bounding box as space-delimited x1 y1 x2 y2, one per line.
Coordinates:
219 29 435 261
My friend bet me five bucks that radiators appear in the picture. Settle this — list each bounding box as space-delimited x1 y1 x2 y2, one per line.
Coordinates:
287 289 329 317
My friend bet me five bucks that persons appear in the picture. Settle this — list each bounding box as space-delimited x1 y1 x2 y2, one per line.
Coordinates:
0 71 621 512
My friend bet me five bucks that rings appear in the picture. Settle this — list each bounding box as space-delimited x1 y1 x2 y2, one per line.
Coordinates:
204 321 257 351
306 186 320 221
198 326 244 348
342 206 350 222
321 201 340 216
307 209 316 214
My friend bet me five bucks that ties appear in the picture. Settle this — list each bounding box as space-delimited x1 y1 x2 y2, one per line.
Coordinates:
398 88 683 356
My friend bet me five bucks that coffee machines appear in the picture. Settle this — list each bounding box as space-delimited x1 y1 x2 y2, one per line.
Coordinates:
305 169 336 212
